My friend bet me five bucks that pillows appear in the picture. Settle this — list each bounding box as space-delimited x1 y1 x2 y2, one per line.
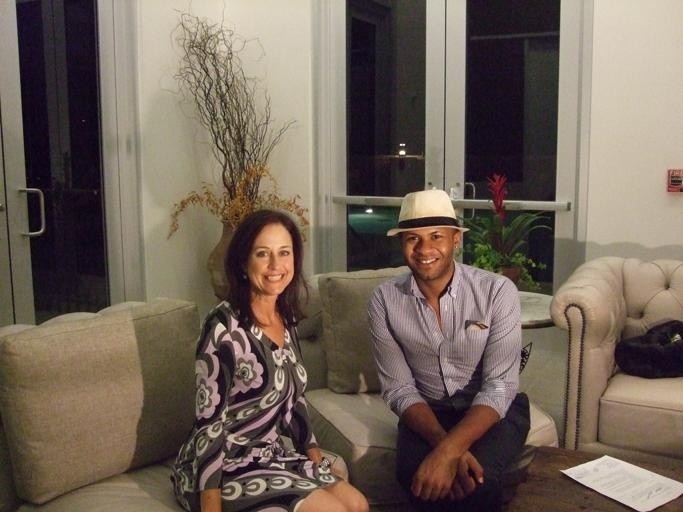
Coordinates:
318 273 404 394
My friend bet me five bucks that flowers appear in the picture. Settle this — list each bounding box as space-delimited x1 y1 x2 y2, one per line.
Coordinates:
455 171 554 296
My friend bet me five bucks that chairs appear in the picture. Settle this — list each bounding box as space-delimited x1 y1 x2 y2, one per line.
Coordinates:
0 296 351 511
548 253 683 468
301 264 560 499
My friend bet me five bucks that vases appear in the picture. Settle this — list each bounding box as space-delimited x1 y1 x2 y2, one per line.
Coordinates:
492 264 521 290
207 217 256 298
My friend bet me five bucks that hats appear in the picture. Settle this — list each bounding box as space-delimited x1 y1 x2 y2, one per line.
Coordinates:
385 187 471 238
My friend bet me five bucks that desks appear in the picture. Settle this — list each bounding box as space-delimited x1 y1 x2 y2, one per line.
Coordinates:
512 287 568 450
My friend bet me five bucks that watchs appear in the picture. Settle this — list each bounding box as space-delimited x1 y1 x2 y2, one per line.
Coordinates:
320 456 331 468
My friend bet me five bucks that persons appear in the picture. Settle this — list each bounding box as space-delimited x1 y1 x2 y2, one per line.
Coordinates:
171 210 370 512
368 189 530 512
